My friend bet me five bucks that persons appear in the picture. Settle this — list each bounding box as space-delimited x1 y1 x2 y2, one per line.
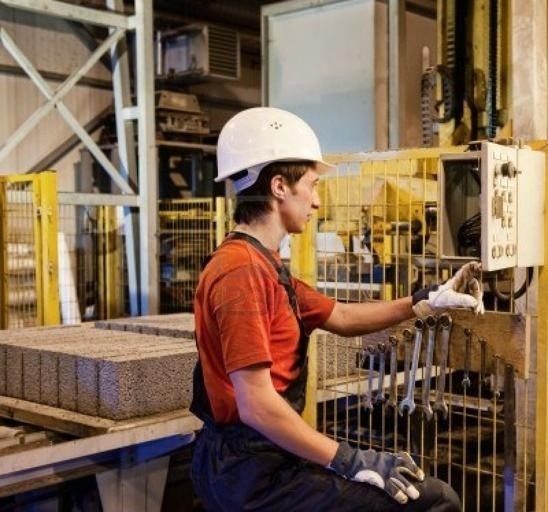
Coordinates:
189 106 487 512
403 217 431 298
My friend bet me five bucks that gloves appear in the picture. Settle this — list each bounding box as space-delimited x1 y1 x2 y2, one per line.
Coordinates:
412 261 485 318
325 441 425 504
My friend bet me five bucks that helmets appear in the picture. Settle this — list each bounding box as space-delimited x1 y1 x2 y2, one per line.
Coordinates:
213 107 338 183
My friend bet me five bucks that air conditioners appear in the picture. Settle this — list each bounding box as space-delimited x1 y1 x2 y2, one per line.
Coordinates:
162 27 243 86
